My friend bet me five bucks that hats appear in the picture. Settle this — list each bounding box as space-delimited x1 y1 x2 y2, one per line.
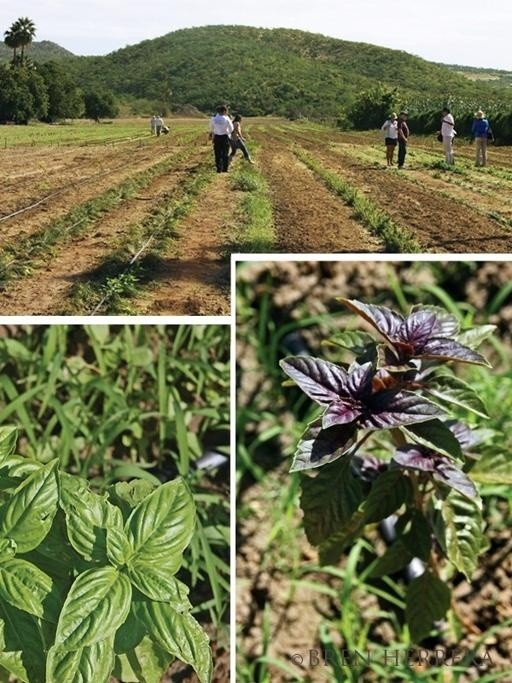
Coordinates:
388 111 408 119
474 110 486 119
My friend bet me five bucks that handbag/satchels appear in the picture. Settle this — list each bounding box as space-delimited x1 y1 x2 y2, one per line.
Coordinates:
437 133 443 142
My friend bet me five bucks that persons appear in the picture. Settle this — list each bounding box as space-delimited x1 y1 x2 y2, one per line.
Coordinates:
381 111 399 166
439 105 456 166
150 115 156 135
207 103 256 174
154 114 164 137
395 109 411 170
470 110 490 169
